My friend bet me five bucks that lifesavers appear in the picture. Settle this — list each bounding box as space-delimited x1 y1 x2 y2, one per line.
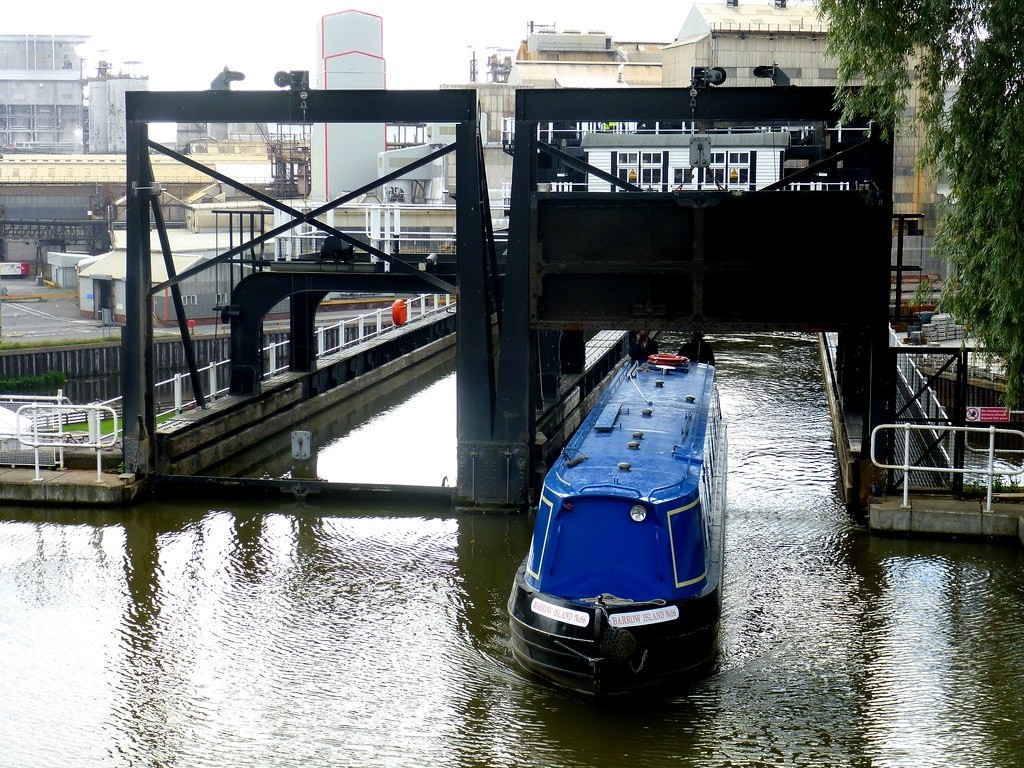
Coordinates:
647 353 689 365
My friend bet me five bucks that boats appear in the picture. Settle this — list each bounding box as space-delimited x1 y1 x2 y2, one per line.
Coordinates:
507 353 729 698
